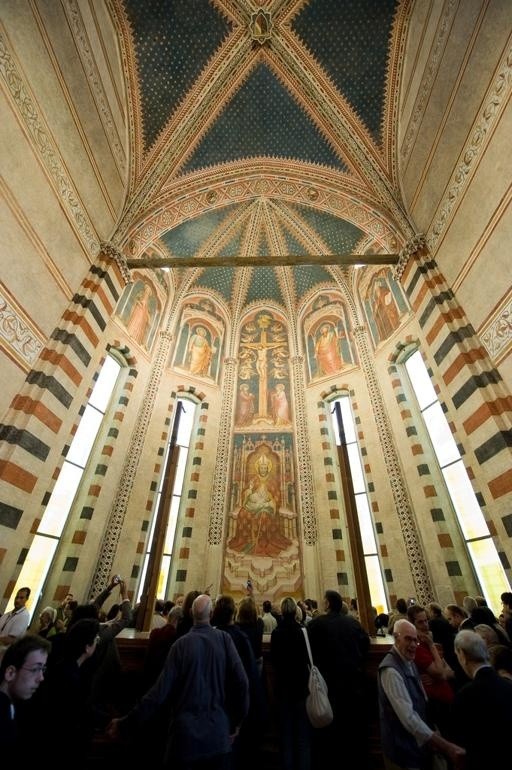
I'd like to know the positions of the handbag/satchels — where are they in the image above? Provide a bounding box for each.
[305,665,334,727]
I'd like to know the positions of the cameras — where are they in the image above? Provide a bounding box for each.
[114,576,122,582]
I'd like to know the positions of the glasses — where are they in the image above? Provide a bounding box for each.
[396,632,420,646]
[21,665,46,675]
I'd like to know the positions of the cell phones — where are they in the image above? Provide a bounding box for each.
[247,580,252,589]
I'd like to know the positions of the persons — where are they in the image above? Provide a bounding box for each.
[372,281,401,341]
[125,283,152,346]
[228,455,292,557]
[187,327,211,377]
[313,324,343,376]
[237,384,255,426]
[270,384,289,426]
